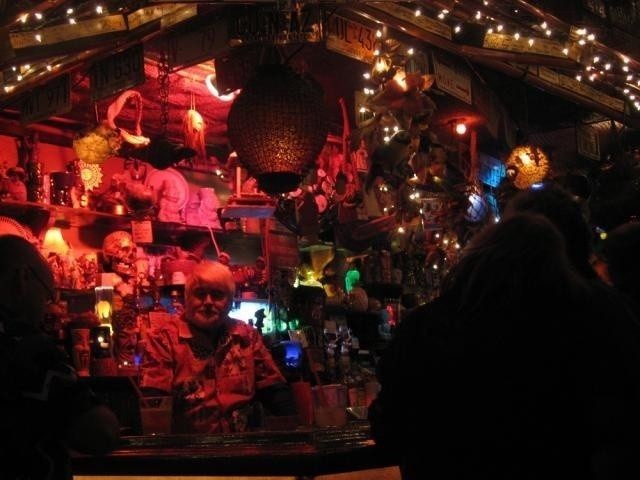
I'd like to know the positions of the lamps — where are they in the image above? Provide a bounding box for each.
[227,45,328,195]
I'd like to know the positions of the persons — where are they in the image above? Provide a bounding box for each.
[135,256,287,431]
[0,233,123,480]
[367,154,639,480]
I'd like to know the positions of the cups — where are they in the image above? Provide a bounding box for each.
[313,385,346,429]
[139,395,173,436]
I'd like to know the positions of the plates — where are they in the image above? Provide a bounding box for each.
[144,167,189,212]
